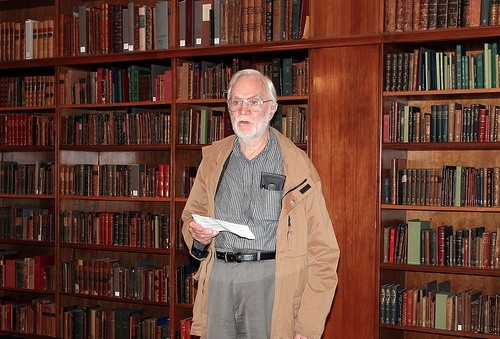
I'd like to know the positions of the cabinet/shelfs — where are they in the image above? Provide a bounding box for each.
[0,0,500,339]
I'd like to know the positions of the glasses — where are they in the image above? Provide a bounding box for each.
[227,97,273,112]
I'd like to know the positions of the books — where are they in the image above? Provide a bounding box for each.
[0,0,308,339]
[379,0,500,339]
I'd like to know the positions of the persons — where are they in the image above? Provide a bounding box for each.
[180,69,340,339]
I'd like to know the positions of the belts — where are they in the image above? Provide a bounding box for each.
[214,250,275,262]
